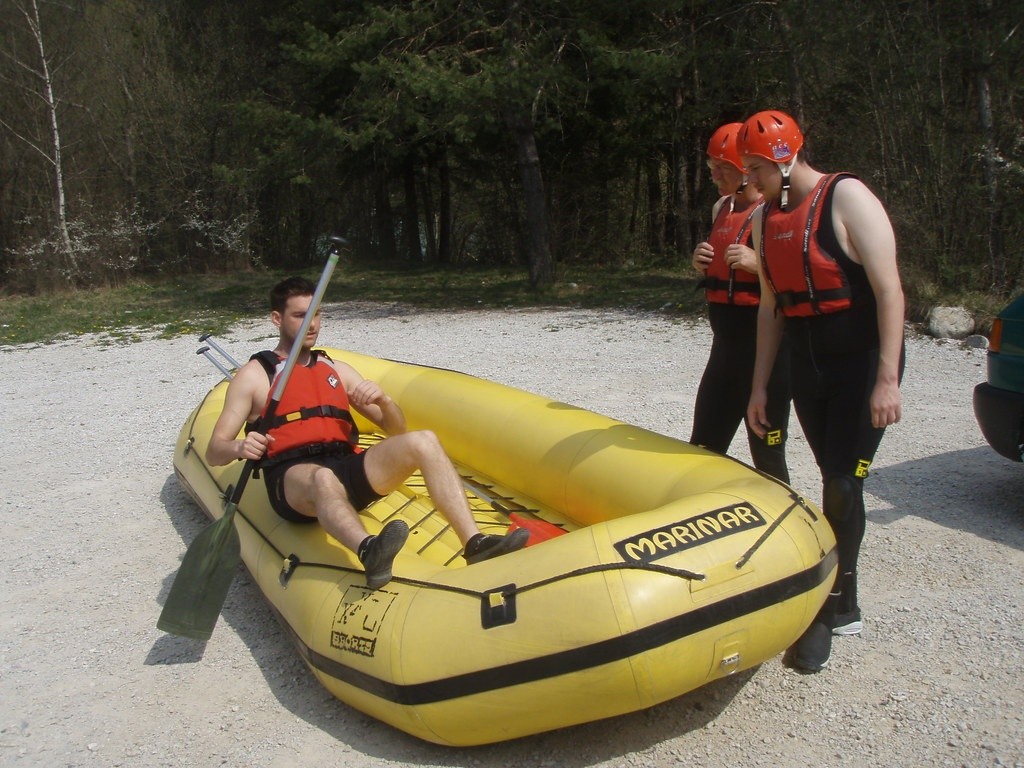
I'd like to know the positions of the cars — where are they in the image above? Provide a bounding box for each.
[974,290,1024,462]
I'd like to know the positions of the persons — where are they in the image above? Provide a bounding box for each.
[735,109,908,636]
[204,277,531,591]
[689,120,791,487]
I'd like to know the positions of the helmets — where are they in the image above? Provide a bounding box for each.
[737,110,804,162]
[707,122,750,174]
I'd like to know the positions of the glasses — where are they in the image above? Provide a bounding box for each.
[706,160,735,172]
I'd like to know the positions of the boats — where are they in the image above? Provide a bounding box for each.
[174,347,839,748]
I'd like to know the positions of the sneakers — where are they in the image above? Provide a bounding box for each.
[461,527,530,566]
[360,520,409,591]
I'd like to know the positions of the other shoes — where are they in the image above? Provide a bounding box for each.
[793,612,831,672]
[830,605,863,634]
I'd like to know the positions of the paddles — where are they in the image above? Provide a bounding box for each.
[195,328,573,550]
[155,234,351,644]
